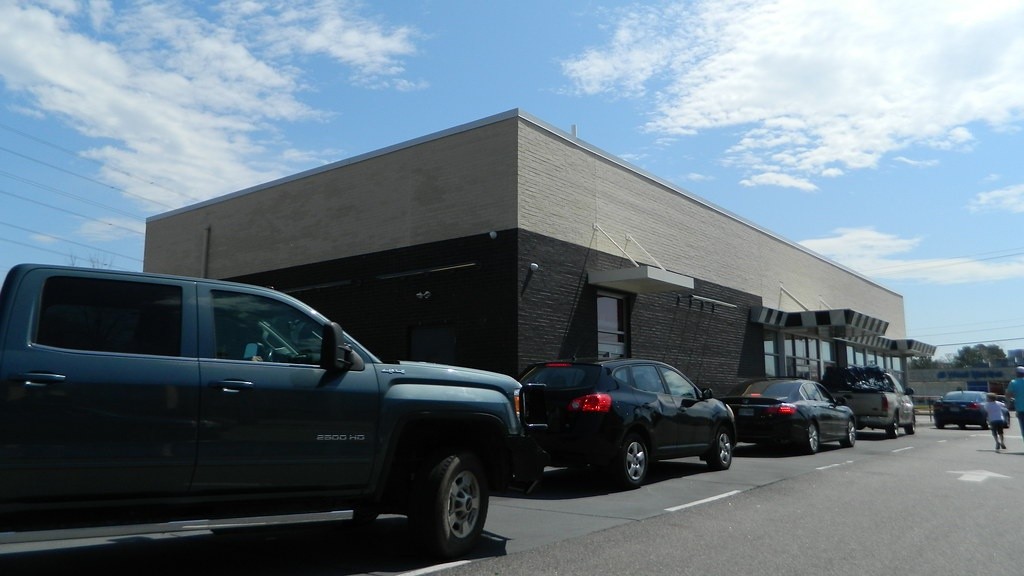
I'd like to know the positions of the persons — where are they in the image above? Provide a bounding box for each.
[983,393,1009,450]
[1006,366,1024,443]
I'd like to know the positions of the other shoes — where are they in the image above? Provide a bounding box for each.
[995,442,1001,449]
[1000,443,1007,449]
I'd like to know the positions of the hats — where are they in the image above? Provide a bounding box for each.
[1015,366,1024,373]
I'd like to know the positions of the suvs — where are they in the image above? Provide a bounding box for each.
[516,350,739,492]
[0,262,528,561]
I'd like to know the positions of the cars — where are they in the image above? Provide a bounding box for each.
[932,387,1015,430]
[831,372,916,440]
[712,376,857,456]
